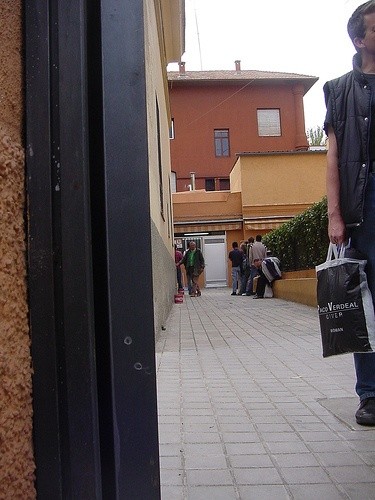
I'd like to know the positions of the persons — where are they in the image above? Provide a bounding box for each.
[323,0,375,427]
[228,236,254,295]
[253,258,282,299]
[176,241,205,297]
[174,244,185,293]
[246,234,267,296]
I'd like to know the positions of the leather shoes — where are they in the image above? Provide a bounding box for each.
[355,397,375,424]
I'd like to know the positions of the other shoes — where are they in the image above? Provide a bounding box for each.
[191,294,195,297]
[253,295,262,299]
[238,293,241,295]
[242,293,246,296]
[231,292,236,295]
[197,290,201,296]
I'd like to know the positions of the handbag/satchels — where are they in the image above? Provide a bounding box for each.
[315,239,375,357]
[241,263,246,273]
[264,284,273,298]
